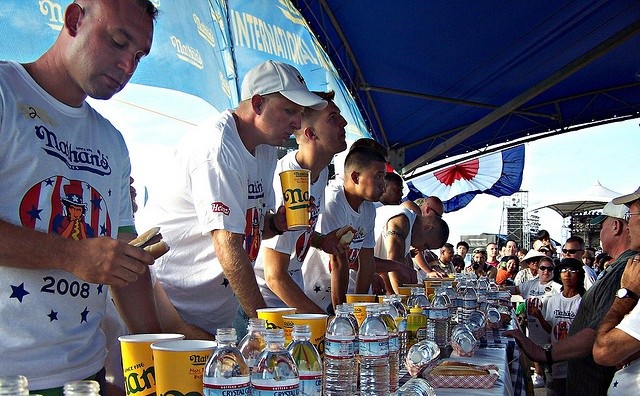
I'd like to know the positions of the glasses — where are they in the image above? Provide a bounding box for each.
[625,210,640,220]
[561,244,583,254]
[559,268,578,274]
[539,266,554,271]
[474,250,485,254]
[429,207,442,218]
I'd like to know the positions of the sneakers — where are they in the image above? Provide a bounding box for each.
[531,372,545,387]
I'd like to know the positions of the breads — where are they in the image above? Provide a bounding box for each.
[339,230,354,248]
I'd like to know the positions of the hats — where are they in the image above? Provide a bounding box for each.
[241,60,329,111]
[554,259,585,280]
[473,248,487,257]
[520,249,547,263]
[590,201,630,225]
[612,187,640,204]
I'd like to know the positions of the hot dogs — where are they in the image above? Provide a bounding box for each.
[123,227,170,260]
[432,361,489,374]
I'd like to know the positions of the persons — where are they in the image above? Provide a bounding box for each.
[99,175,215,395]
[349,137,389,160]
[455,240,470,259]
[398,199,423,216]
[514,249,542,286]
[372,172,404,209]
[302,145,386,314]
[536,229,551,243]
[505,255,520,281]
[135,59,329,334]
[231,89,348,346]
[502,201,638,395]
[517,249,528,262]
[529,257,587,346]
[466,247,498,278]
[538,244,553,257]
[485,243,500,267]
[505,239,518,256]
[347,202,449,295]
[592,186,640,396]
[582,250,595,269]
[496,242,506,263]
[412,195,449,279]
[451,254,465,273]
[519,257,563,299]
[596,252,609,267]
[0,0,163,396]
[600,255,613,271]
[562,236,597,291]
[438,242,457,274]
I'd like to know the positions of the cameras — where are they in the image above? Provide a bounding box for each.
[473,262,482,270]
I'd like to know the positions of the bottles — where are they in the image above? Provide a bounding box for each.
[375,302,401,391]
[383,293,407,369]
[323,303,359,396]
[201,328,250,396]
[250,328,300,395]
[236,317,269,372]
[451,324,476,353]
[285,324,322,396]
[360,305,392,396]
[407,286,430,352]
[408,339,441,365]
[388,379,440,396]
[430,272,501,349]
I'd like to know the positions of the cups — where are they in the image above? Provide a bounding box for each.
[279,168,313,231]
[282,313,329,355]
[149,340,218,396]
[117,332,186,396]
[255,307,297,329]
[377,294,410,306]
[345,293,376,331]
[398,286,412,296]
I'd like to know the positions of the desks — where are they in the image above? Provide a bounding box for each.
[395,350,514,396]
[478,313,528,396]
